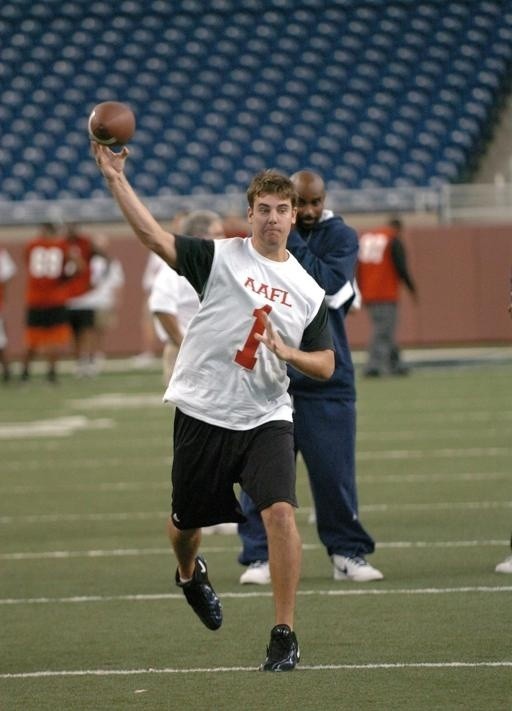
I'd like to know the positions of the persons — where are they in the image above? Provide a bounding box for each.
[494,529,512,575]
[143,211,237,535]
[1,214,120,383]
[89,141,335,673]
[237,171,383,585]
[357,219,418,376]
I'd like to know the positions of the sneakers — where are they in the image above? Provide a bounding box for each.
[172,550,226,634]
[238,560,275,586]
[256,623,305,677]
[330,552,386,586]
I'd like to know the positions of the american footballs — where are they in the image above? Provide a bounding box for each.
[87,101,136,147]
